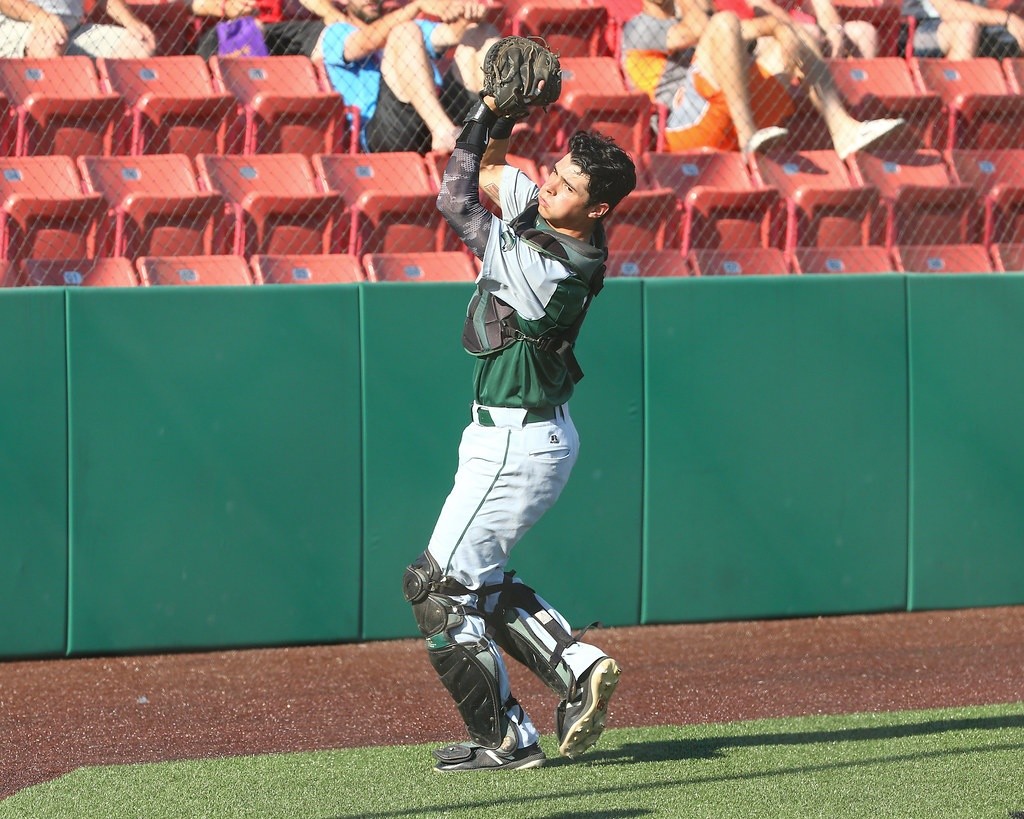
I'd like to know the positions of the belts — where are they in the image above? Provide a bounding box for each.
[478,404,563,428]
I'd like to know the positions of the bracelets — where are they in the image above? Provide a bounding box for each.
[1002,9,1013,29]
[216,1,229,16]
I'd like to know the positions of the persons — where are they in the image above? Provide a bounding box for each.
[401,54,638,772]
[901,0,1024,60]
[619,0,909,157]
[171,1,529,153]
[0,1,157,59]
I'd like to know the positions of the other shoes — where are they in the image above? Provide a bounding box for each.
[742,125,791,162]
[832,117,907,160]
[430,127,462,157]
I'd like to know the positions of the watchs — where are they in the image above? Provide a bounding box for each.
[833,22,847,33]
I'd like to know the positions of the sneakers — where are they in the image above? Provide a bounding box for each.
[432,737,548,774]
[558,657,622,760]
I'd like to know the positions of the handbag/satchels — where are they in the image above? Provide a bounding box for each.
[216,8,267,58]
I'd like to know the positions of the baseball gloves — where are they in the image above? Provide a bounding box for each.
[480,33,562,121]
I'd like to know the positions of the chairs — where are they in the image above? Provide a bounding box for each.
[0,0,1024,288]
